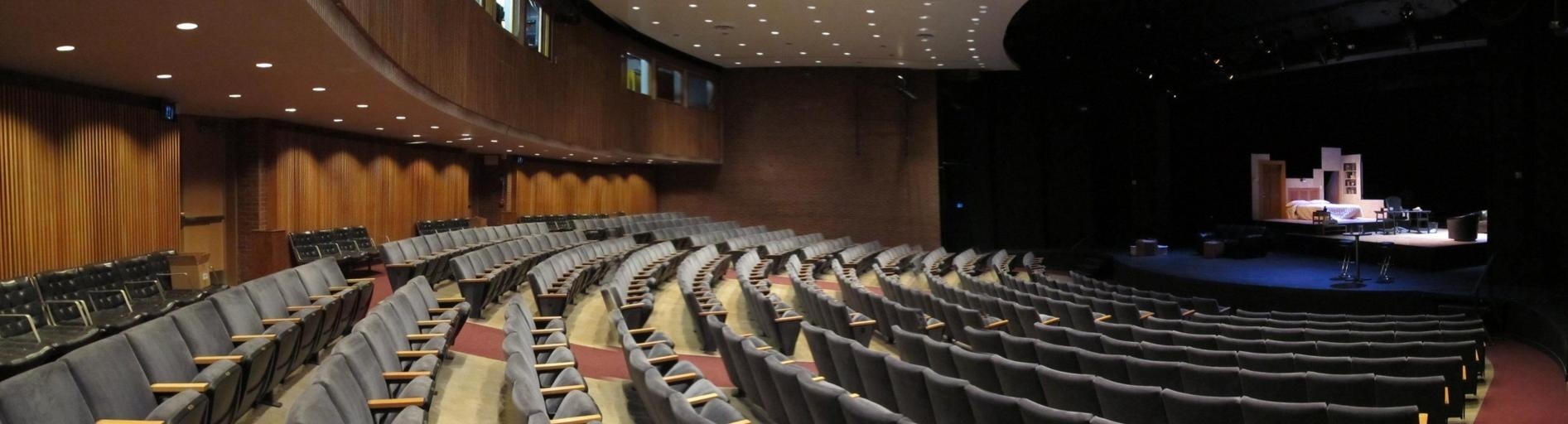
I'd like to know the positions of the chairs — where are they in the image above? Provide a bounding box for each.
[0,210,1489,424]
[1385,196,1410,233]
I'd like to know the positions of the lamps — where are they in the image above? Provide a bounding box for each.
[1301,177,1306,182]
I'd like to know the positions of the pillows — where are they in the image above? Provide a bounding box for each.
[1285,200,1308,207]
[1310,199,1330,203]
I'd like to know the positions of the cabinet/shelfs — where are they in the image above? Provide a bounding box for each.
[1343,163,1357,195]
[1259,160,1286,220]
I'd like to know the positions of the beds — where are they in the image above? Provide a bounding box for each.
[1286,203,1361,220]
